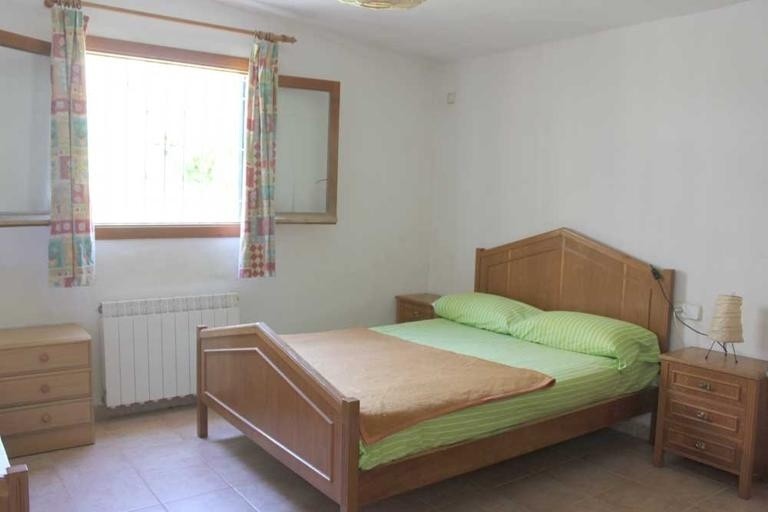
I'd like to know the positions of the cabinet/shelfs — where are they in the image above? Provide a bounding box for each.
[0,324,94,458]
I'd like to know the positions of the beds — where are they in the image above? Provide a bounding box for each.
[195,226,677,510]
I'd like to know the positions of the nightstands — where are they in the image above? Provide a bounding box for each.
[652,346,768,499]
[394,293,440,320]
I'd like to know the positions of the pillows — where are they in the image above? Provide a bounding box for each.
[431,293,540,335]
[510,310,660,370]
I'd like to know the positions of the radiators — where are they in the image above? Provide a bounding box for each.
[99,292,241,409]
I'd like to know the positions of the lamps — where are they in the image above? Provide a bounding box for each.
[705,293,744,364]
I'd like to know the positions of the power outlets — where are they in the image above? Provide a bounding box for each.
[673,304,702,320]
